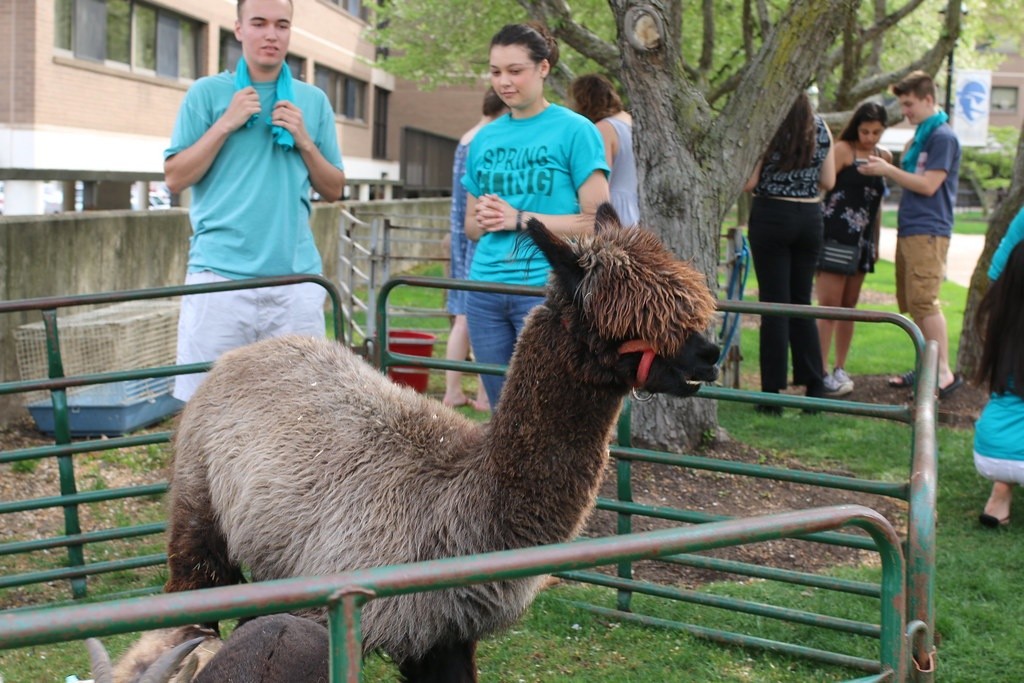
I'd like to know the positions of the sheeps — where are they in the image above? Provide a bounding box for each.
[81,613,330,683]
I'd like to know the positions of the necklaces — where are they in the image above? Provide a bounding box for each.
[873,147,877,158]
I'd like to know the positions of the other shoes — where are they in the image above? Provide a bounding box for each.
[802,408,821,414]
[754,404,784,415]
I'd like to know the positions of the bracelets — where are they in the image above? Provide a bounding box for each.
[516,209,526,232]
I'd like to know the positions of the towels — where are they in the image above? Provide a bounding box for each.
[900,110,948,173]
[234,53,296,152]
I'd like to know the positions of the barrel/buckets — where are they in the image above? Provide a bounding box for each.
[374,331,435,392]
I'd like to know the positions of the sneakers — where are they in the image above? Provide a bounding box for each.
[833,368,854,387]
[822,372,852,396]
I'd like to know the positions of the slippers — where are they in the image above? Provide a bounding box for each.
[453,395,488,413]
[889,370,916,387]
[979,514,1010,528]
[939,374,963,399]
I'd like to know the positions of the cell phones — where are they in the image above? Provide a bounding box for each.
[855,159,868,167]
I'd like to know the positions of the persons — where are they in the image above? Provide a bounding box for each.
[571,69,641,237]
[163,2,348,402]
[855,68,961,400]
[742,84,838,416]
[967,196,1024,529]
[815,102,895,400]
[438,84,512,416]
[462,24,614,428]
